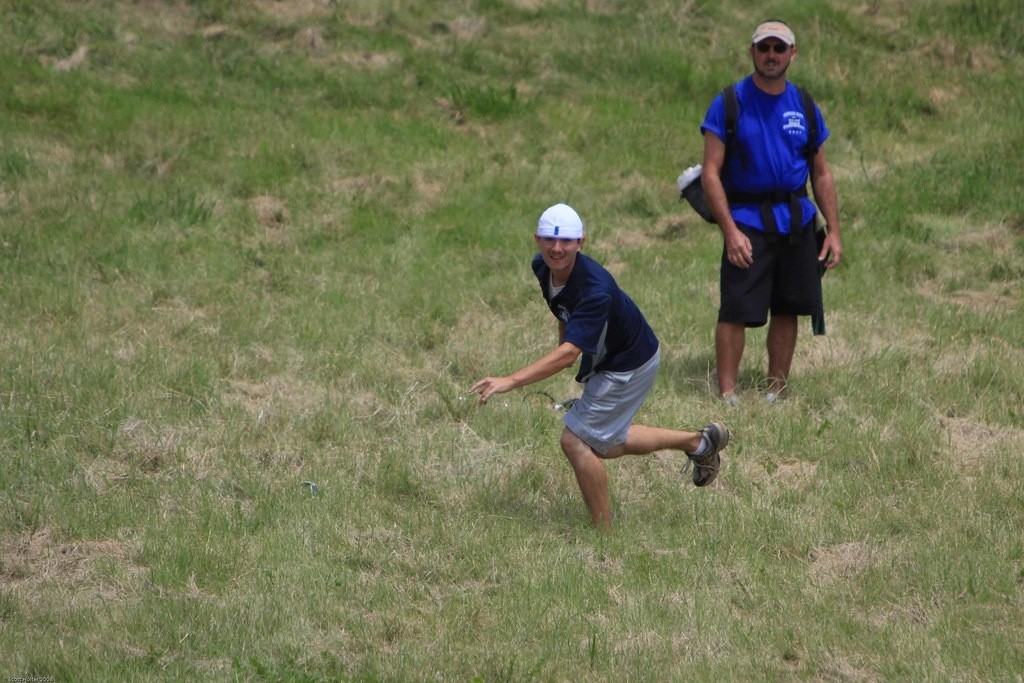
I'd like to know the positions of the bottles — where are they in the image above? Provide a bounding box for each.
[677,163,705,193]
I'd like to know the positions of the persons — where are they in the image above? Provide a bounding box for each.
[701,19,843,412]
[468,202,729,538]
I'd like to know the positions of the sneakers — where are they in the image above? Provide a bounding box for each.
[767,394,785,400]
[686,420,729,486]
[719,391,738,405]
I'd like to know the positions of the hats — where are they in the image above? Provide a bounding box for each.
[537,205,583,239]
[752,22,795,46]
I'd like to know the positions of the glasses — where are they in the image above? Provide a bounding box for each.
[753,44,792,53]
